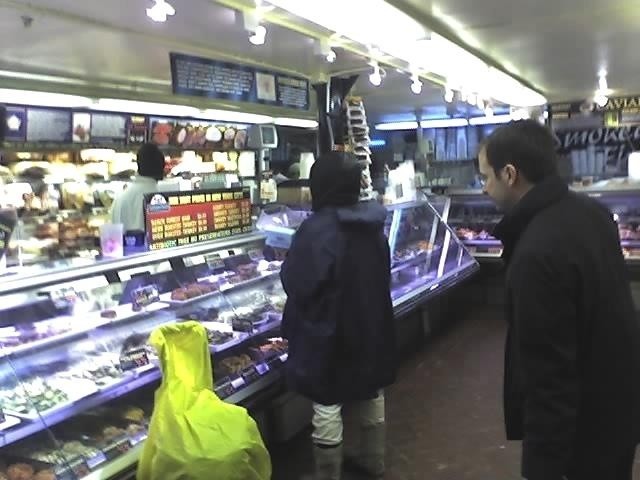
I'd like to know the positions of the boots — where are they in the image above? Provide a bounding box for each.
[344,423,386,477]
[299,445,343,480]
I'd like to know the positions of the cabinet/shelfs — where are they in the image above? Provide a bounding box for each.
[0,196,482,480]
[441,187,639,304]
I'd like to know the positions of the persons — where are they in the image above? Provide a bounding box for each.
[475,117,640,478]
[276,144,397,480]
[108,141,166,236]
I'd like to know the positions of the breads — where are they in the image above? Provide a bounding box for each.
[0,462,55,480]
[171,284,216,300]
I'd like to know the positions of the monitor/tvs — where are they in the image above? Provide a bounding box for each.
[247,125,278,149]
